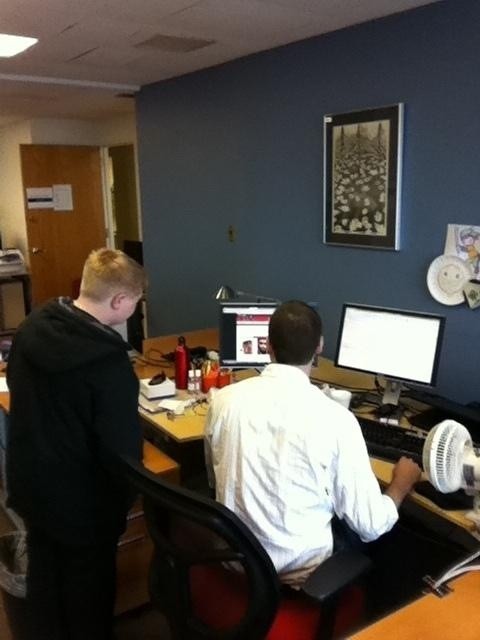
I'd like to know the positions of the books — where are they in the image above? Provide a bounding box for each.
[139,394,178,413]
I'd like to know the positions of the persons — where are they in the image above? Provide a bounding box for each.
[257,336,268,355]
[6,248,148,640]
[203,300,422,630]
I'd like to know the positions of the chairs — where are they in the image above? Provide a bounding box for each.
[110,448,389,629]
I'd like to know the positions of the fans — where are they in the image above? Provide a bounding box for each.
[421,418,480,533]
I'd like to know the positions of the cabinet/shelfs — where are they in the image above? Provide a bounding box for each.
[1,272,33,335]
[115,489,181,616]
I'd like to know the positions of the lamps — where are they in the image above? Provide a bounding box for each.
[210,282,284,314]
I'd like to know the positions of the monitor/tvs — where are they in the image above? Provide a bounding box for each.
[335,303,447,389]
[219,302,318,368]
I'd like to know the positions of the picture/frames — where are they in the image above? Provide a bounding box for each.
[318,100,405,252]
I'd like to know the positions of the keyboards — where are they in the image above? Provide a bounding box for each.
[355,417,428,472]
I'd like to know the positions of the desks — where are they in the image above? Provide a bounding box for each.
[1,390,180,484]
[344,569,480,640]
[125,326,480,543]
